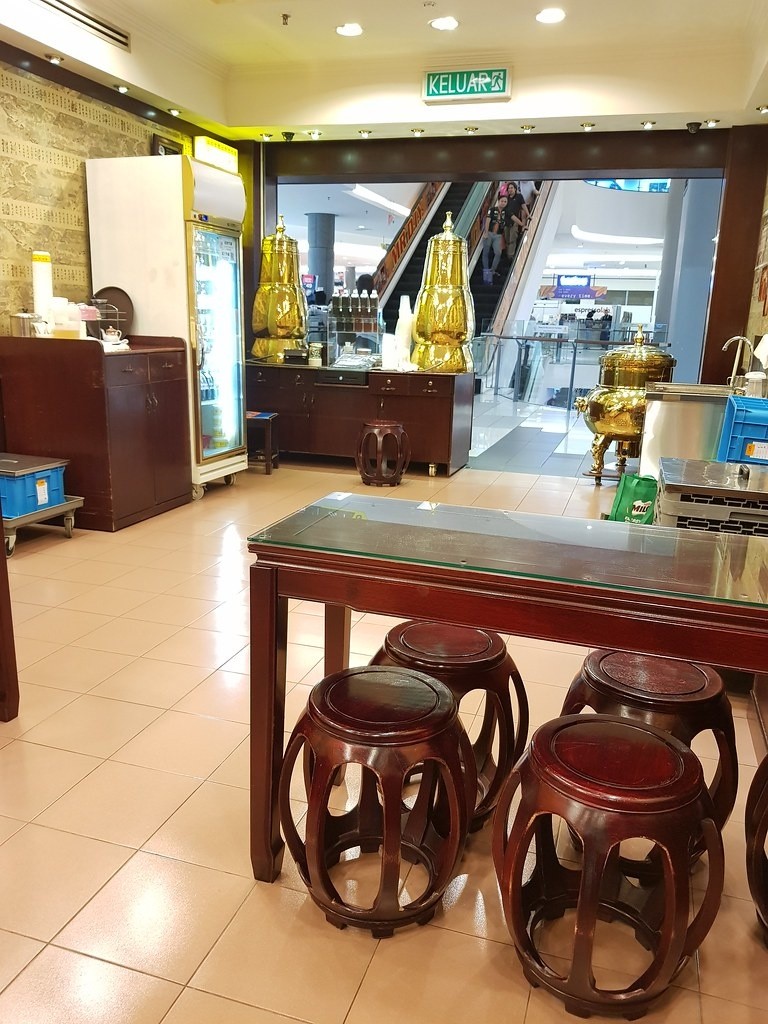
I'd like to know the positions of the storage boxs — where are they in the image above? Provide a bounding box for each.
[0,466,67,519]
[715,395,768,466]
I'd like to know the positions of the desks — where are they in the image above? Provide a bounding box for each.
[651,458,768,537]
[248,492,768,885]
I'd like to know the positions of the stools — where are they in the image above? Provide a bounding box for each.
[558,649,739,879]
[246,411,279,476]
[278,666,477,939]
[356,420,412,487]
[745,752,768,950]
[492,715,727,1022]
[368,620,529,834]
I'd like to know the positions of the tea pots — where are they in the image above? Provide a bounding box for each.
[100,326,122,342]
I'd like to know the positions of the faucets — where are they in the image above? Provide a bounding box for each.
[721,333,753,374]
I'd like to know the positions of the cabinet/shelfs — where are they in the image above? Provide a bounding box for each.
[246,358,481,478]
[0,334,191,533]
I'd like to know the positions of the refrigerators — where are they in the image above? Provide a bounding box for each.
[85,155,249,500]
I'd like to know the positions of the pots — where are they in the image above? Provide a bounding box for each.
[9,308,46,338]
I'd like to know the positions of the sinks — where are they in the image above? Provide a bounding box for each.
[658,386,733,394]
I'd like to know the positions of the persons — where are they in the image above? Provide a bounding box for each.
[600,311,612,351]
[582,312,594,349]
[356,274,373,295]
[482,180,542,285]
[314,291,326,305]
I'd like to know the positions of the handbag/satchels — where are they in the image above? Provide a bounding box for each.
[606,474,658,527]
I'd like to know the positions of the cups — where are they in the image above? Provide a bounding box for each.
[727,376,745,386]
[90,299,108,318]
[357,348,372,355]
[398,295,411,370]
